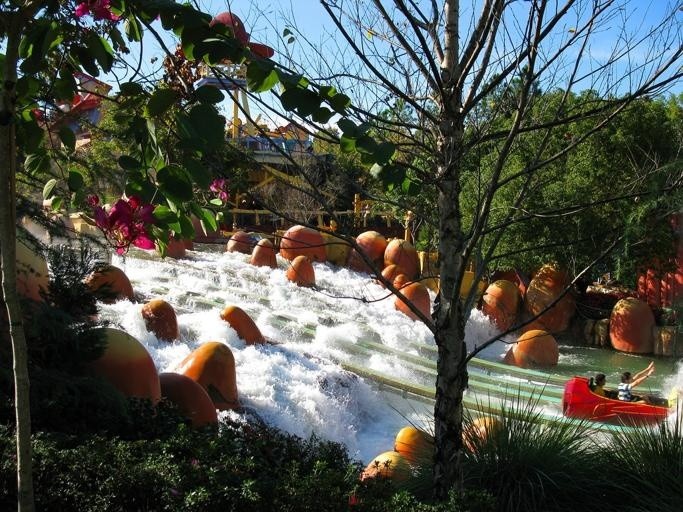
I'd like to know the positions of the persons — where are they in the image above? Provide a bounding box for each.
[616,360,657,405]
[592,370,608,397]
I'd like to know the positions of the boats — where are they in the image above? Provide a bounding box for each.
[560,375,682,431]
